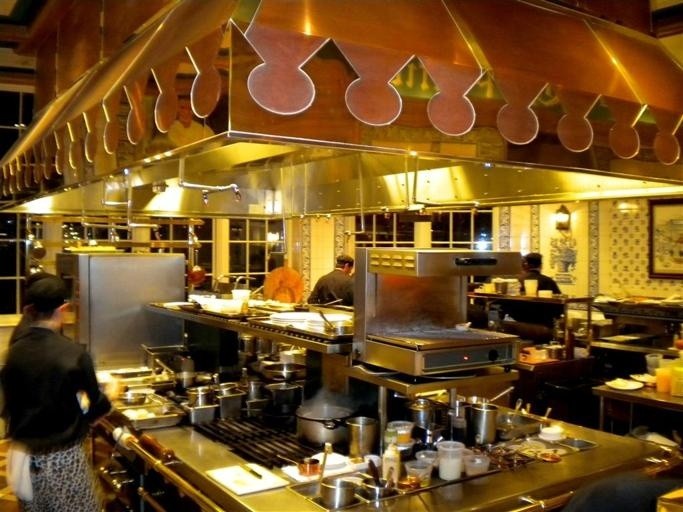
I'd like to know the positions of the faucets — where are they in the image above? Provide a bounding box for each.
[211,272,238,293]
[228,274,256,295]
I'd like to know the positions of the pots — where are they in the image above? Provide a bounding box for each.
[292,405,354,450]
[174,372,203,389]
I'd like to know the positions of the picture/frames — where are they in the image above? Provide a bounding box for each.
[646,196,682,281]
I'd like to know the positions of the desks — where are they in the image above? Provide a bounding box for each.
[591,377,682,433]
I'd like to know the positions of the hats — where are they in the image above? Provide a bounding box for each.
[337,255,354,265]
[522,253,542,268]
[28,278,65,306]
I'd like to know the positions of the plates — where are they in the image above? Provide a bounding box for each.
[603,373,656,392]
[270,311,351,330]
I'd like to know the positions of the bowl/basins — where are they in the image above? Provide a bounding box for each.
[114,392,148,405]
[262,382,302,414]
[261,364,305,382]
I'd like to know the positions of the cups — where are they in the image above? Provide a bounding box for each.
[450,398,499,445]
[184,383,239,405]
[231,290,250,306]
[437,483,462,504]
[391,420,413,444]
[483,283,493,293]
[344,415,375,458]
[321,477,393,510]
[645,351,682,397]
[413,221,432,249]
[405,441,491,486]
[495,282,506,294]
[524,279,537,297]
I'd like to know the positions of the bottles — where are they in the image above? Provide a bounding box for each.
[381,421,401,488]
[238,334,281,362]
[238,366,249,385]
[136,435,175,462]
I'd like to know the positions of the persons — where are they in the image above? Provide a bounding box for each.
[7,272,69,348]
[168,94,214,148]
[0,273,112,512]
[502,252,564,342]
[306,254,355,309]
